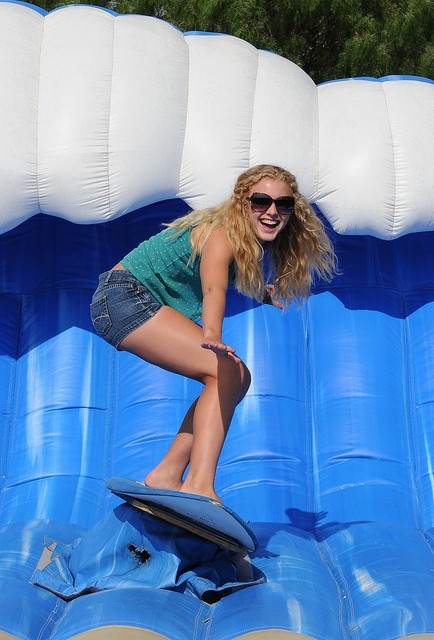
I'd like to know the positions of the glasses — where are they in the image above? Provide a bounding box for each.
[243,192,298,215]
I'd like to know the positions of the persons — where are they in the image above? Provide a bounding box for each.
[89,164,343,505]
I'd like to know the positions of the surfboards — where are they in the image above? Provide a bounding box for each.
[106,479,259,553]
